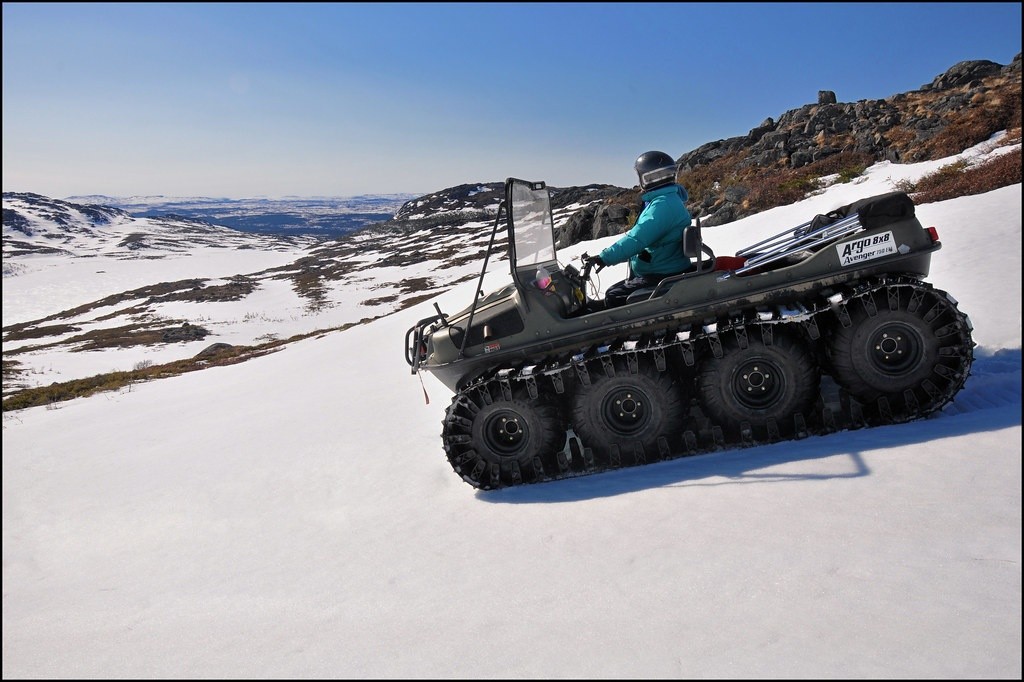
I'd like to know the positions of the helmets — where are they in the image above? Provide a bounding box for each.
[634,150,678,191]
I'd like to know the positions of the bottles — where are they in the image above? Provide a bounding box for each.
[536,264,556,297]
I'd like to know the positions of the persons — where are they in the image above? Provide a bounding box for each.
[582,151,691,309]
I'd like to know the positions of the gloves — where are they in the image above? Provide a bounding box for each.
[581,254,606,274]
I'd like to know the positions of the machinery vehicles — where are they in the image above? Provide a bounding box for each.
[403,176,979,492]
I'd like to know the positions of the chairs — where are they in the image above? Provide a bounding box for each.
[626,217,717,306]
